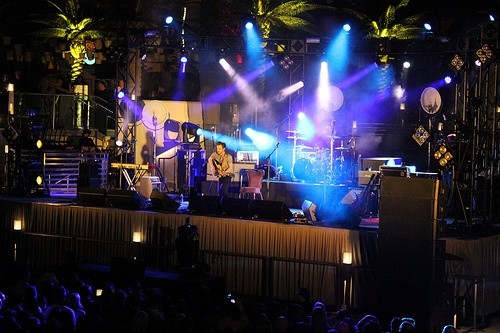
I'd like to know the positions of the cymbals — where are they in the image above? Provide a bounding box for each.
[286,130,303,133]
[287,136,307,140]
[335,147,349,149]
[325,135,341,138]
[287,149,300,150]
[293,145,313,149]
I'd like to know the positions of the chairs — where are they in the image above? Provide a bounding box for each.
[239,169,266,199]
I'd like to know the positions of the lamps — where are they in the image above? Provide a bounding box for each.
[446,53,465,72]
[161,13,175,28]
[115,140,124,147]
[117,90,131,103]
[215,51,225,64]
[181,120,202,150]
[433,145,455,169]
[87,53,95,65]
[244,15,253,30]
[424,20,435,31]
[279,55,296,71]
[410,124,431,146]
[140,47,147,60]
[474,44,498,65]
[180,50,189,63]
[164,119,182,141]
[489,11,500,22]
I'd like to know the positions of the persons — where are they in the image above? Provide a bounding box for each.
[441,325,458,333]
[93,81,116,135]
[79,129,98,179]
[114,80,129,97]
[208,141,234,198]
[0,262,417,333]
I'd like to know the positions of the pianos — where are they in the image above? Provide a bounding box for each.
[111,163,157,191]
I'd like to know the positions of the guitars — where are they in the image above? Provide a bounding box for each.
[350,174,380,217]
[211,164,235,179]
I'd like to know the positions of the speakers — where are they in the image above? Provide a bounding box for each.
[189,195,224,215]
[77,187,108,205]
[150,192,181,212]
[104,191,143,210]
[223,197,253,218]
[252,199,294,221]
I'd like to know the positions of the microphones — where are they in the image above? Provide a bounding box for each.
[275,142,279,148]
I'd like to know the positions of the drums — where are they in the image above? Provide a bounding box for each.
[292,157,319,179]
[316,148,330,159]
[302,153,317,158]
